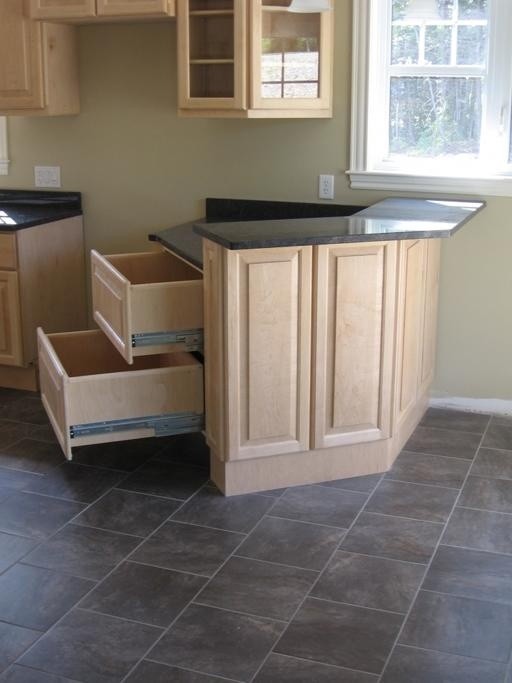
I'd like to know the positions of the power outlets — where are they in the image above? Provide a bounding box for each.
[318,173,336,199]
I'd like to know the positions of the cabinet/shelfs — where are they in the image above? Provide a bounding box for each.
[171,0,335,124]
[1,0,80,117]
[1,186,91,397]
[27,0,177,28]
[28,197,486,501]
